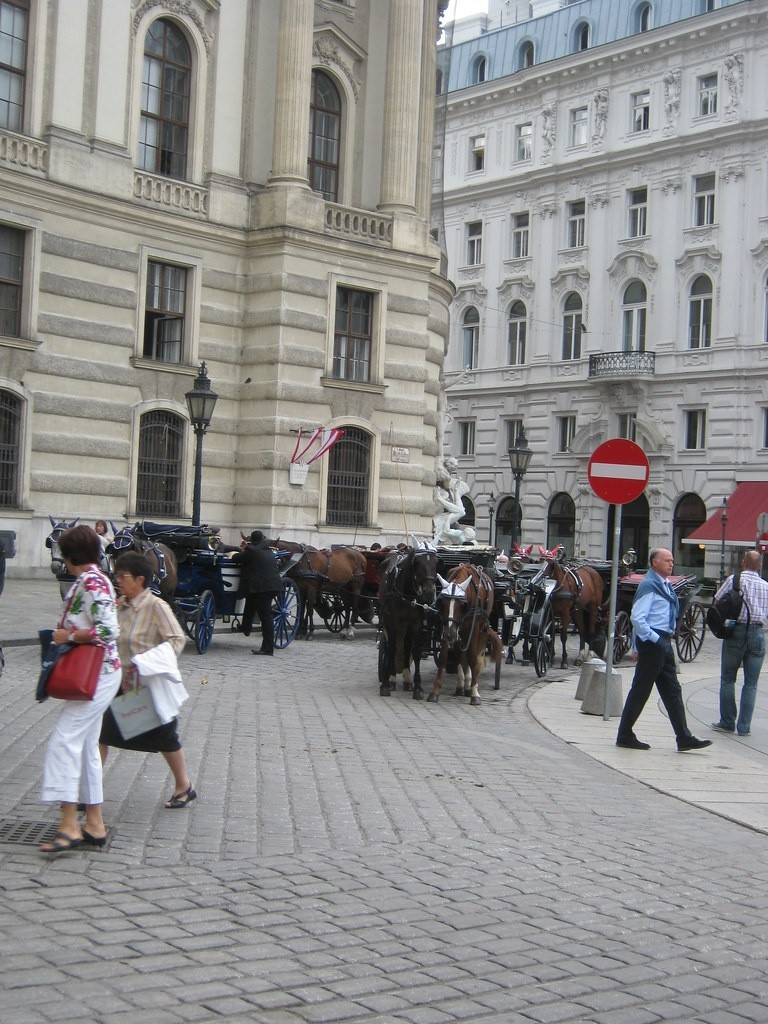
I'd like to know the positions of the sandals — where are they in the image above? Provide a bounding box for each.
[165,784,197,809]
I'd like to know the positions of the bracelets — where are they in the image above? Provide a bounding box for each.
[68,633,74,643]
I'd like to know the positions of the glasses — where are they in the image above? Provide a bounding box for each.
[113,571,135,579]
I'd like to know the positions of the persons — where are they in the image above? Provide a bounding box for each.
[541,104,553,145]
[616,549,713,751]
[210,527,242,552]
[663,71,678,121]
[59,551,198,813]
[498,555,508,563]
[592,91,607,138]
[232,531,284,656]
[724,56,739,98]
[711,550,768,736]
[436,476,478,545]
[40,524,123,851]
[95,520,113,543]
[350,543,381,623]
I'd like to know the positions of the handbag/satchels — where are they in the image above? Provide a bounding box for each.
[35,625,105,703]
[109,668,164,740]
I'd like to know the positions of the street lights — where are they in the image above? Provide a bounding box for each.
[716,494,731,587]
[506,424,535,562]
[486,491,498,547]
[183,360,219,526]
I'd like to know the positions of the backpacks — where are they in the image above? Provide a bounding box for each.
[706,572,751,640]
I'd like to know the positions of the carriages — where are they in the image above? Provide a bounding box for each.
[199,522,707,707]
[44,514,302,655]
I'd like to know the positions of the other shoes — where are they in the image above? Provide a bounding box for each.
[738,730,752,736]
[711,721,735,735]
[237,622,250,637]
[251,649,273,656]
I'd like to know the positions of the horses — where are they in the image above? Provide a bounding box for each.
[46,513,114,602]
[531,545,604,670]
[375,534,438,699]
[266,537,366,641]
[239,529,318,554]
[510,542,540,579]
[427,563,494,704]
[104,520,177,608]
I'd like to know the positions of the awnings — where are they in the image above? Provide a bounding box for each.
[681,481,768,547]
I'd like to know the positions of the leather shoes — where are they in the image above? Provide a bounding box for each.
[617,738,650,748]
[678,738,712,751]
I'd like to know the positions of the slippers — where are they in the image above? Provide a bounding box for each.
[41,827,87,852]
[78,827,106,846]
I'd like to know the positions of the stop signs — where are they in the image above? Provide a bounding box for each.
[587,438,650,505]
[757,532,768,555]
